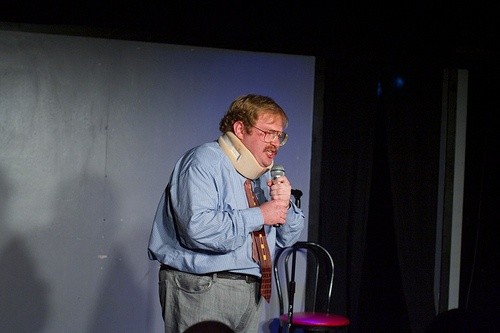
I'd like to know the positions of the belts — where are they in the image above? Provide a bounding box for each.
[165,265,261,283]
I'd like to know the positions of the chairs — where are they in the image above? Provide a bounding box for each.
[273,239,350,333]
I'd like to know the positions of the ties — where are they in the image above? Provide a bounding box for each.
[244,178,273,304]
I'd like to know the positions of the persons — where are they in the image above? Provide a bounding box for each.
[147,95,305,333]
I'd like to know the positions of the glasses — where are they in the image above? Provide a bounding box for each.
[250,124,289,146]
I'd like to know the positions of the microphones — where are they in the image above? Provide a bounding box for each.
[271,166,285,227]
